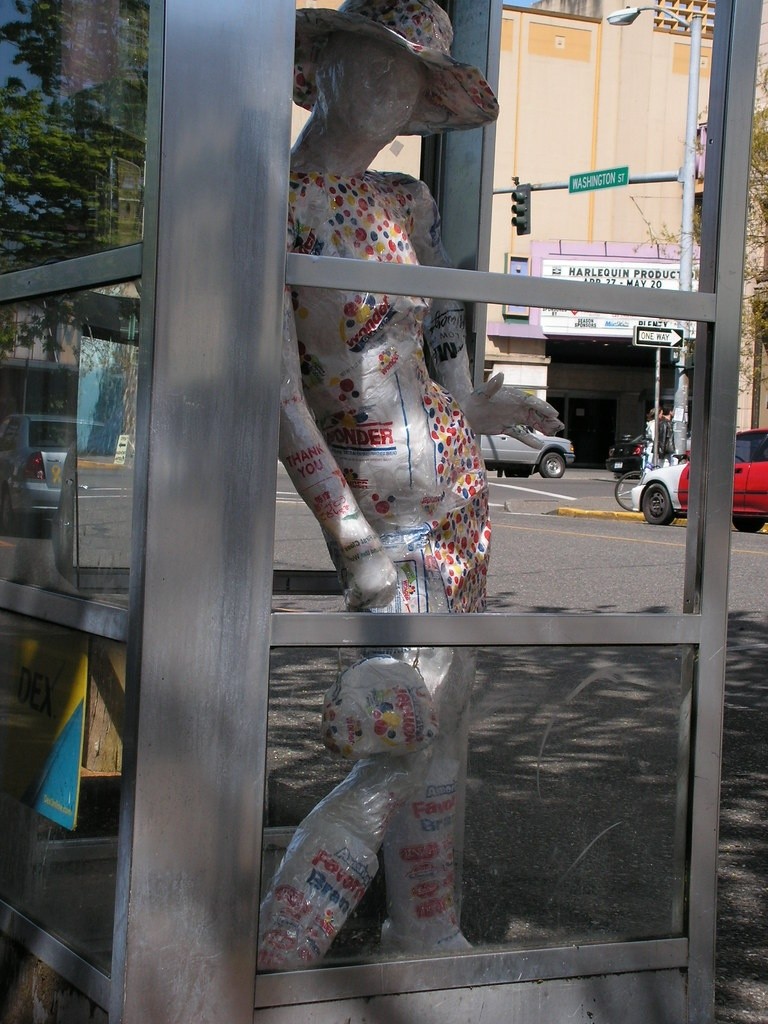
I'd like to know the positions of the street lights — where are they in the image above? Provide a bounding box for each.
[606,3,703,456]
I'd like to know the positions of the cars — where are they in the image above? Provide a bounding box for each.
[605,431,649,478]
[0,413,109,539]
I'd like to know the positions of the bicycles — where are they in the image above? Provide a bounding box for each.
[614,436,688,513]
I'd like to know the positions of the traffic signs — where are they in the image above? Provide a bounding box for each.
[631,324,686,350]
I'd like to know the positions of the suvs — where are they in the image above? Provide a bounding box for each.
[480,424,576,478]
[631,427,768,534]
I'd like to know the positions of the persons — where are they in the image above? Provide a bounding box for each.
[642,404,675,472]
[254,0,566,970]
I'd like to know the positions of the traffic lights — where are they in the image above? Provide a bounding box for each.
[510,182,531,236]
